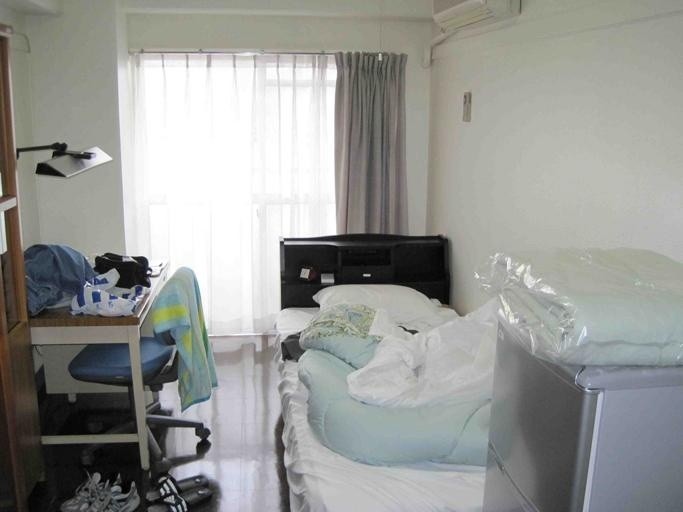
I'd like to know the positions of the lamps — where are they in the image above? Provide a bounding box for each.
[16,141,113,180]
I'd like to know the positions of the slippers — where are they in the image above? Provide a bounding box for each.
[145,474,211,512]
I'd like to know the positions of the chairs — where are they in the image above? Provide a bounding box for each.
[67,265,210,478]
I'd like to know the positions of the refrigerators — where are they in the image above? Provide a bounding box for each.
[482,316,683,511]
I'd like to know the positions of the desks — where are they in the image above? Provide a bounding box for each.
[28,259,171,471]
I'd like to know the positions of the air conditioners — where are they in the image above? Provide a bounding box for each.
[433,0,521,31]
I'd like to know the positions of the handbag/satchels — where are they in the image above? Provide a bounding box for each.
[94,252,152,287]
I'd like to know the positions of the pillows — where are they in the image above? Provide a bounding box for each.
[311,284,440,315]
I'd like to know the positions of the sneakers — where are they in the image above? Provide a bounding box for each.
[88,479,141,511]
[60,470,122,511]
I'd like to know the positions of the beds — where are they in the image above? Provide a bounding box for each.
[278,232,485,512]
